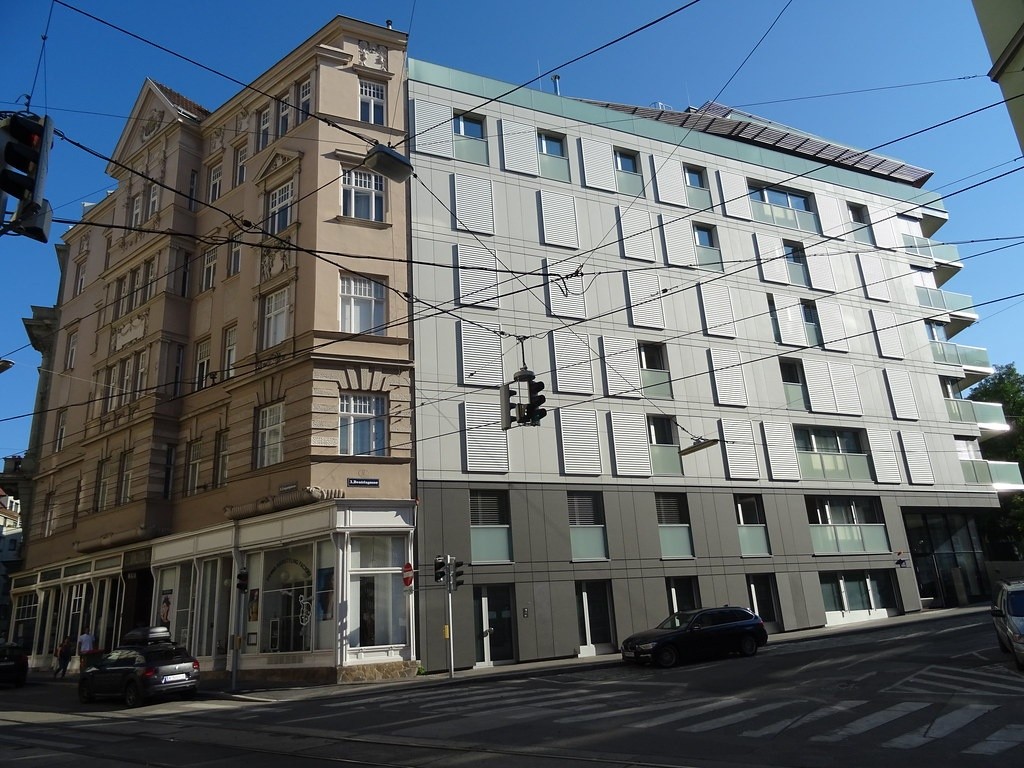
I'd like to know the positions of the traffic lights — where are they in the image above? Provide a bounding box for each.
[500,384,517,430]
[450,557,464,592]
[528,377,547,426]
[236,571,248,594]
[435,556,446,583]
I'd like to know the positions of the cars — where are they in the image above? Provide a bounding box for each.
[620,606,768,668]
[0,642,28,688]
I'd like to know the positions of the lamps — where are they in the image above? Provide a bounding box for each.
[678,439,721,456]
[363,144,414,185]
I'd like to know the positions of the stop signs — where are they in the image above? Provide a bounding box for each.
[403,564,414,586]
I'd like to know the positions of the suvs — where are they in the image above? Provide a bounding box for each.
[78,639,200,708]
[991,577,1024,671]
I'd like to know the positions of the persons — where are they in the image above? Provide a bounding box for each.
[78,626,96,655]
[52,636,74,681]
[159,597,170,631]
